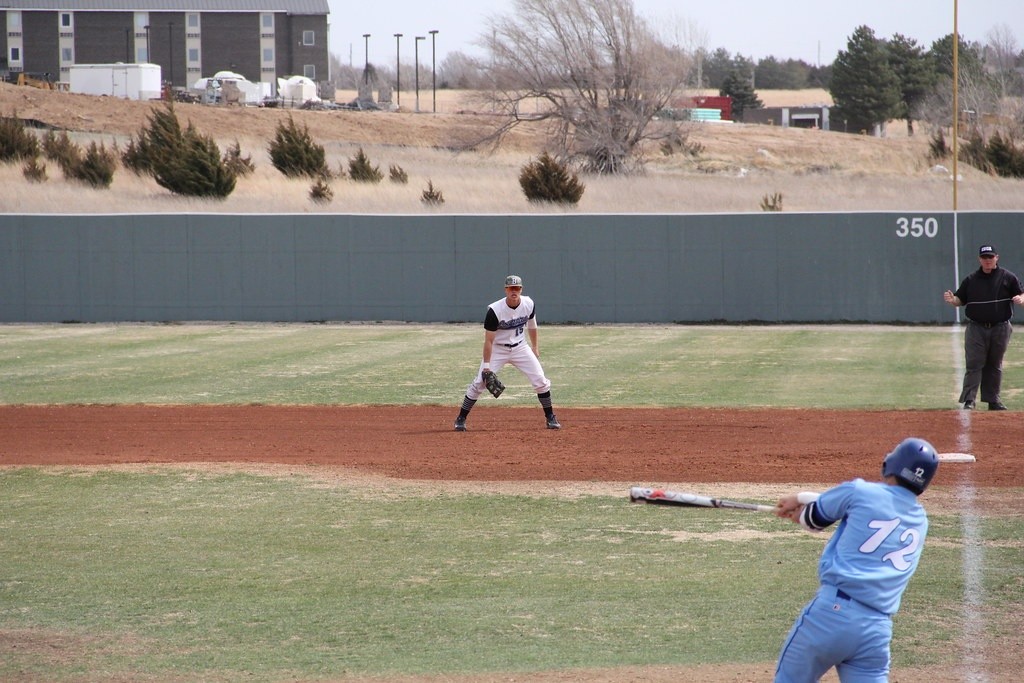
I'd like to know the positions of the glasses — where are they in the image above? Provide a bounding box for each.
[980,255,994,259]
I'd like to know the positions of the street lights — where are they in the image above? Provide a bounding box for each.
[394,33,404,106]
[143,25,152,63]
[415,37,426,112]
[363,34,372,85]
[125,27,132,64]
[167,22,175,86]
[429,30,440,112]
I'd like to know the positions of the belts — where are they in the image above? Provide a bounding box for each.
[971,321,1008,328]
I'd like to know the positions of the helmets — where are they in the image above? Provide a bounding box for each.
[882,438,938,489]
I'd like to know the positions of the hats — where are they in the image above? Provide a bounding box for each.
[504,275,522,288]
[979,243,997,257]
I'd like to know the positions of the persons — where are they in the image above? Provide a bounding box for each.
[943,245,1024,410]
[775,438,938,683]
[454,275,561,431]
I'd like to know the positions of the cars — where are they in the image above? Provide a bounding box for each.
[174,89,222,104]
[259,95,279,107]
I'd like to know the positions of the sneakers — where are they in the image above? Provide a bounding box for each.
[454,417,466,431]
[546,414,560,429]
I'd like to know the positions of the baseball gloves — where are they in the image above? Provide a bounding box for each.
[481,370,506,399]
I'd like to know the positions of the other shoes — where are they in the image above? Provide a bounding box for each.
[964,400,975,409]
[989,403,1007,410]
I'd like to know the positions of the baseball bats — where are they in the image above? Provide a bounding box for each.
[629,485,794,519]
